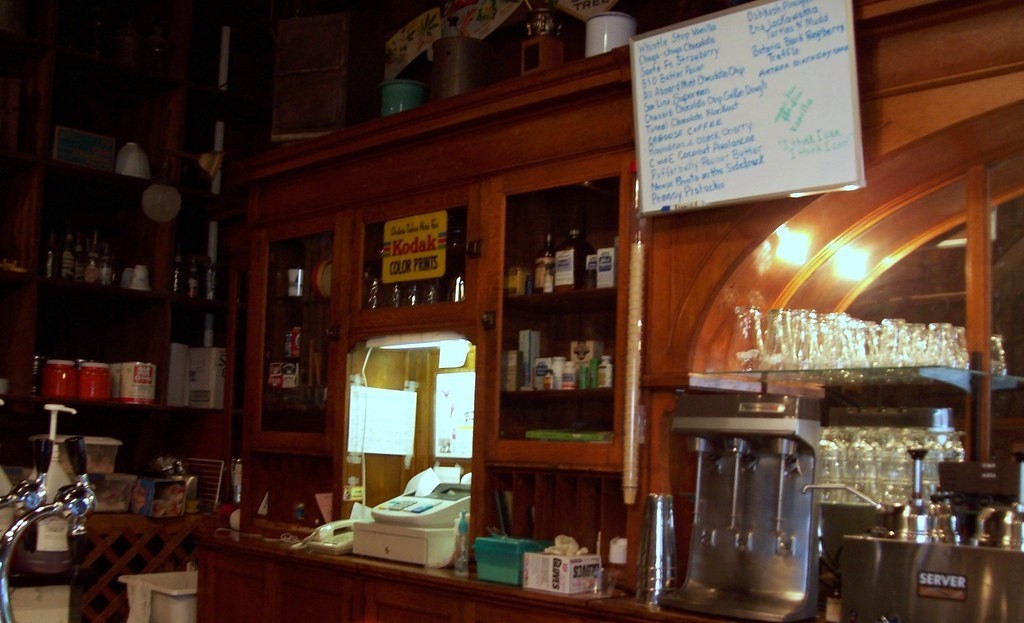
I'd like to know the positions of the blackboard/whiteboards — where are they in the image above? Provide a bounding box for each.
[627,0,867,218]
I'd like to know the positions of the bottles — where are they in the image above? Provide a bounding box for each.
[46,222,119,285]
[173,256,184,293]
[187,257,199,299]
[455,510,469,576]
[555,225,586,291]
[535,234,556,292]
[272,272,286,297]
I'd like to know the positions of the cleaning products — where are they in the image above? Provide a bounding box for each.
[36,403,84,553]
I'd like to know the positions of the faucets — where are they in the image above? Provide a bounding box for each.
[802,483,883,513]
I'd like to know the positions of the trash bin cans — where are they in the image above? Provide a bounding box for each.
[117,570,198,623]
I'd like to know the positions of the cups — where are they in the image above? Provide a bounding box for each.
[43,360,76,399]
[820,425,964,503]
[378,80,430,116]
[585,12,637,57]
[637,493,684,602]
[128,265,151,290]
[78,363,113,400]
[732,306,1004,384]
[120,268,134,288]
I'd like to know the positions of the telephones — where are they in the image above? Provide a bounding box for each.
[307,520,354,555]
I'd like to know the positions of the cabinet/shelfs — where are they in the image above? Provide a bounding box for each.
[0,0,239,623]
[190,0,1024,623]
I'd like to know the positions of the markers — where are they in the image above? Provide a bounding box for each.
[660,201,702,211]
